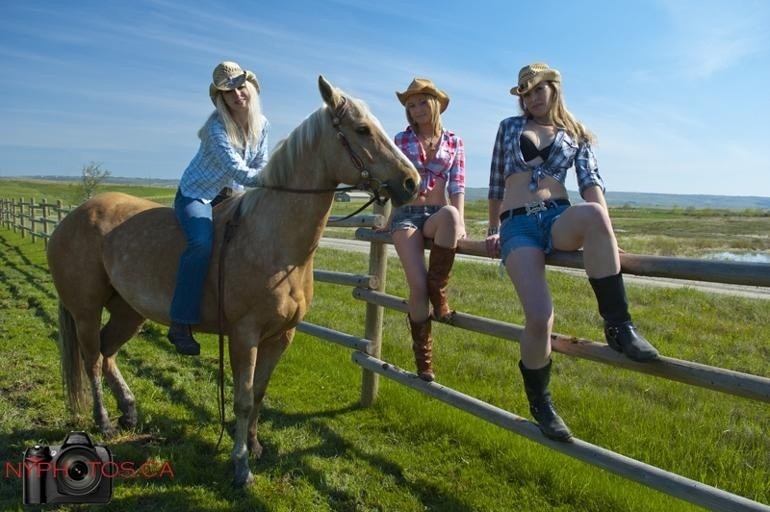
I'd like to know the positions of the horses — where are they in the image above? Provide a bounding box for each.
[44,75,421,494]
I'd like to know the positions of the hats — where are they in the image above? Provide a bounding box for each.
[510,64,561,95]
[396,78,448,114]
[210,61,260,107]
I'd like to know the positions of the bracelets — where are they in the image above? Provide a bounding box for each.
[487,224,498,237]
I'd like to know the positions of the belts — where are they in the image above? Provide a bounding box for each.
[500,198,570,222]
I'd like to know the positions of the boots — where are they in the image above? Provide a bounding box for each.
[518,359,573,441]
[427,244,457,322]
[589,270,659,361]
[408,312,435,381]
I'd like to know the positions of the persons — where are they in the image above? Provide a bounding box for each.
[167,62,269,355]
[486,62,659,440]
[372,79,466,381]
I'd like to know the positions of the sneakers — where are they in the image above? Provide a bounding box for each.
[168,327,200,355]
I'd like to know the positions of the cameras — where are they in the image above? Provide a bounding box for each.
[20,429,114,508]
